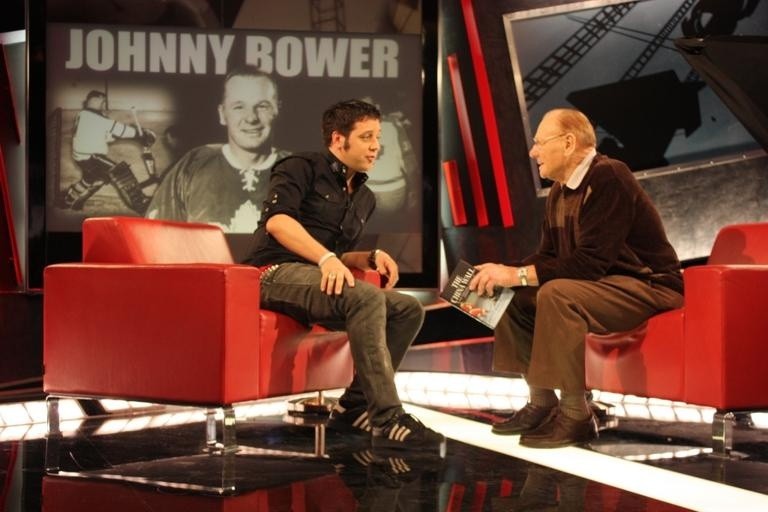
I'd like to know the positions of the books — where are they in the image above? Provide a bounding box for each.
[438,260,516,332]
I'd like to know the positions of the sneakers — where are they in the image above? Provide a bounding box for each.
[372,414,443,450]
[326,402,371,435]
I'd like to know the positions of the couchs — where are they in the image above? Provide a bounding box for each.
[43,218,381,496]
[586,222,766,462]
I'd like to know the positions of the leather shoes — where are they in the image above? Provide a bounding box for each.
[492,403,551,435]
[519,406,600,449]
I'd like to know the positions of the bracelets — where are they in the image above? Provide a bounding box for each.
[368,248,380,270]
[318,252,336,266]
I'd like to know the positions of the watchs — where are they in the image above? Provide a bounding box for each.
[518,266,527,286]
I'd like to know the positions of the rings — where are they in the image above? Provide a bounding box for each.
[328,271,336,279]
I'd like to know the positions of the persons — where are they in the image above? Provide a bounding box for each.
[57,90,156,214]
[352,95,423,244]
[238,98,444,451]
[158,125,205,186]
[145,65,294,234]
[468,108,686,449]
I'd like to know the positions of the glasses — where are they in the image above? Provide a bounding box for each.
[533,135,567,146]
